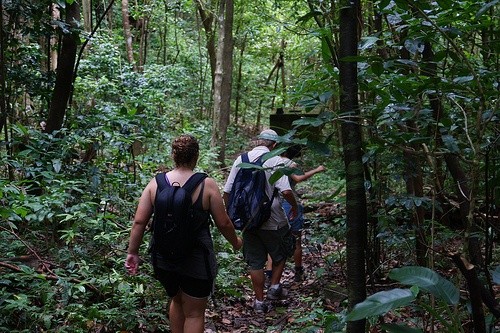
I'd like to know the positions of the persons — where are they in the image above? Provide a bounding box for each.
[123,132,244,333]
[220,130,300,313]
[267,143,327,289]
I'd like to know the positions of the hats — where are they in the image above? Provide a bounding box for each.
[258,129,278,141]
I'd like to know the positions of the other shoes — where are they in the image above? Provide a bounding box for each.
[294,267,304,281]
[266,285,290,301]
[254,298,269,313]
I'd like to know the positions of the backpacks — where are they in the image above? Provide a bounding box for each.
[226,151,277,230]
[153,171,209,256]
